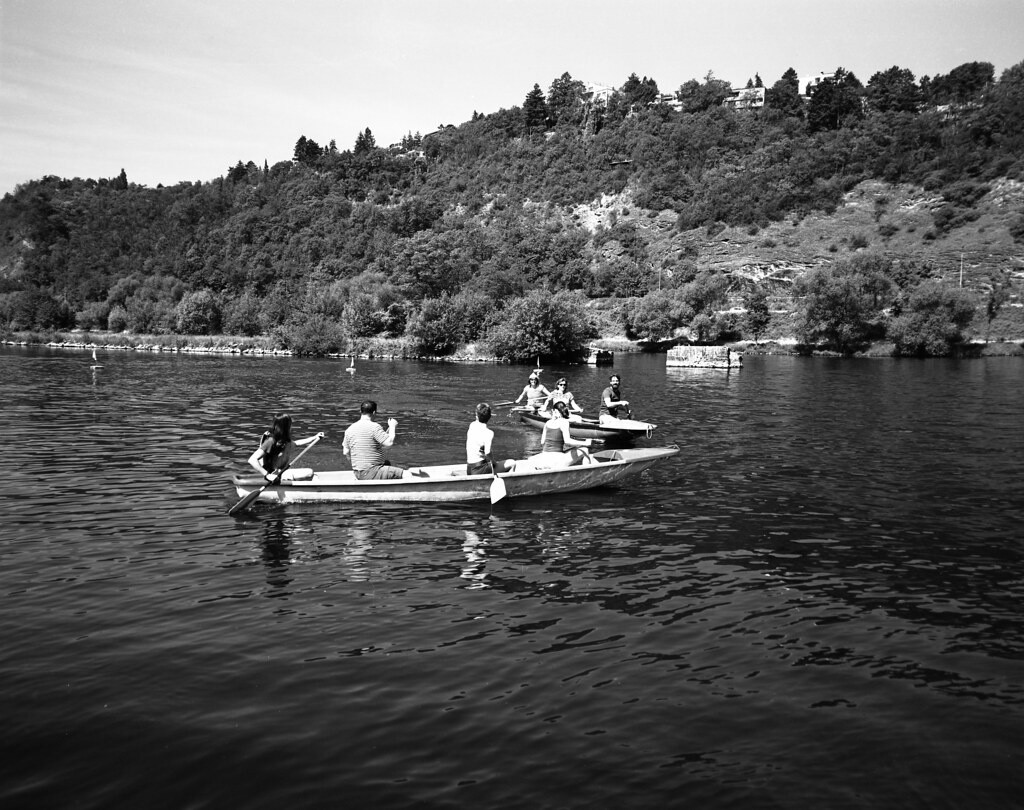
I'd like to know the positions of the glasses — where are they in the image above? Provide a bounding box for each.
[559,384,566,386]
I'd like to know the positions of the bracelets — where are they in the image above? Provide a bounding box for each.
[264,472,270,480]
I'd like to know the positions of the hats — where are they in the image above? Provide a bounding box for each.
[529,372,539,378]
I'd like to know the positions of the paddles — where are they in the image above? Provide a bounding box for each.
[564,446,574,453]
[475,403,507,505]
[625,404,632,420]
[492,396,548,406]
[229,433,322,516]
[386,427,389,433]
[510,405,580,413]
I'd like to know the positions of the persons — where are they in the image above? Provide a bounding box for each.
[514,373,584,422]
[599,374,630,424]
[341,400,412,480]
[540,401,593,468]
[247,412,325,481]
[464,403,517,475]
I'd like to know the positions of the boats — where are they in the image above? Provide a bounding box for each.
[225,443,681,508]
[517,403,659,443]
[662,343,744,370]
